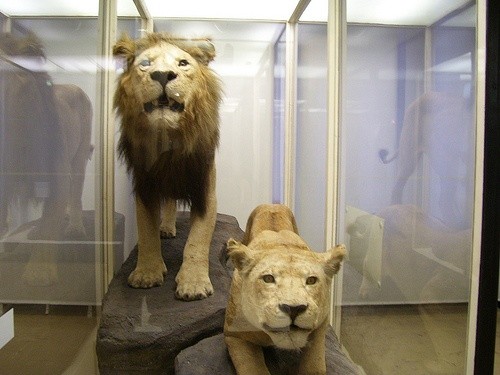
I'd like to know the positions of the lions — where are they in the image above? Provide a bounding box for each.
[111,29,227,301]
[0,30,95,286]
[218,203,348,375]
